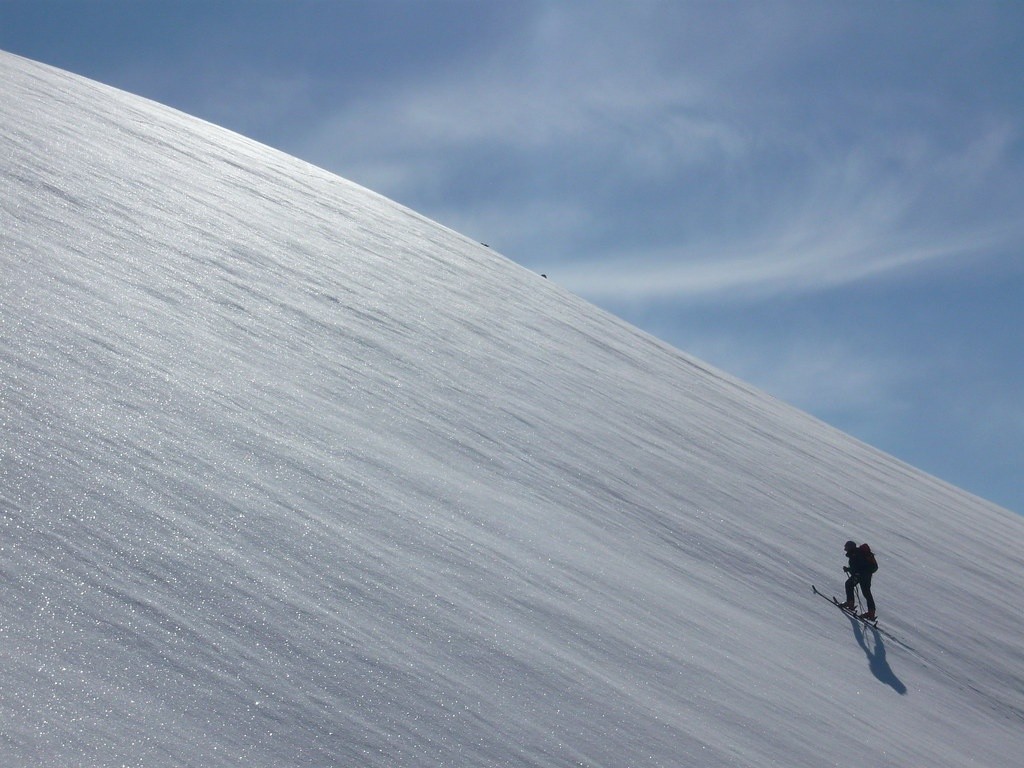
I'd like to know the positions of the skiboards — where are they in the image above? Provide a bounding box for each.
[811,584,878,628]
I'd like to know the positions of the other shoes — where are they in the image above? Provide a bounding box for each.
[863,610,875,618]
[842,600,854,607]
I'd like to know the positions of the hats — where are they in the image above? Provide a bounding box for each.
[845,541,856,550]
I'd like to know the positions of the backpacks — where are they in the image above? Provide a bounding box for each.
[859,543,878,573]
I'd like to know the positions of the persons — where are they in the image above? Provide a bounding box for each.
[841,541,876,619]
